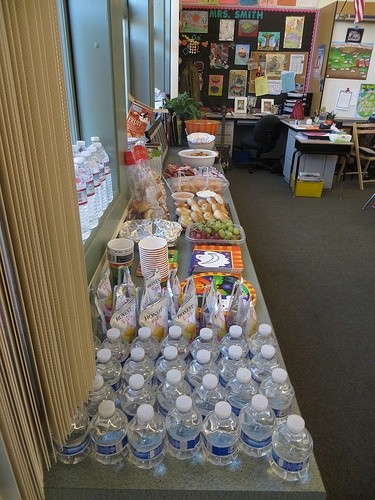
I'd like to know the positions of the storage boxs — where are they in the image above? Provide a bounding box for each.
[290,174,325,197]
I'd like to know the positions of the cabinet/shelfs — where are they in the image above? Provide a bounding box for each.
[215,120,234,159]
[283,128,338,189]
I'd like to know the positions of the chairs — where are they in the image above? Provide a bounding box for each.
[237,115,282,174]
[340,122,375,190]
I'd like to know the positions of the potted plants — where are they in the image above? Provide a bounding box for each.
[326,111,336,125]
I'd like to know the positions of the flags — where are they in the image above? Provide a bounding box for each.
[354,0,364,24]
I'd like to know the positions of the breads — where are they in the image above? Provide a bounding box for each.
[174,193,230,228]
[173,179,221,194]
[133,176,167,220]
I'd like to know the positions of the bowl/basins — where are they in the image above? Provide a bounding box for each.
[184,119,221,136]
[178,149,218,169]
[187,133,216,150]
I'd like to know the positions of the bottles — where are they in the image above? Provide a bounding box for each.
[73,136,114,241]
[52,323,314,481]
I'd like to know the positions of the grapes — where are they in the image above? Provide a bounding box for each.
[189,220,241,241]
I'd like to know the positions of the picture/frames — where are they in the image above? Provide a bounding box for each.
[261,99,274,113]
[235,97,247,113]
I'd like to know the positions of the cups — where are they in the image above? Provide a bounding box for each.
[107,238,134,285]
[138,237,170,291]
[170,192,195,205]
[196,191,216,201]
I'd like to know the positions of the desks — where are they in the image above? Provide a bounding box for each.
[43,147,327,500]
[204,111,333,133]
[288,135,354,200]
